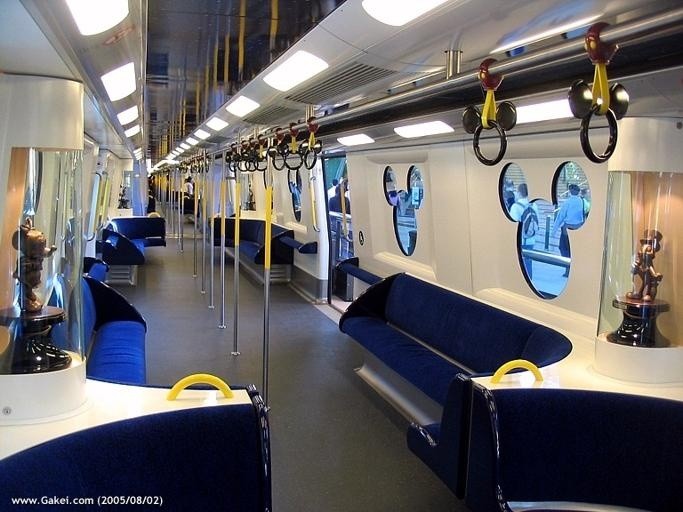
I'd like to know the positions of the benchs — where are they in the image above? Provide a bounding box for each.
[207,216,294,286]
[338,268,574,449]
[147,196,156,212]
[176,192,199,214]
[33,273,259,396]
[97,216,166,288]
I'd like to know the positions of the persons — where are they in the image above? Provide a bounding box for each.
[147,174,195,213]
[291,170,590,282]
